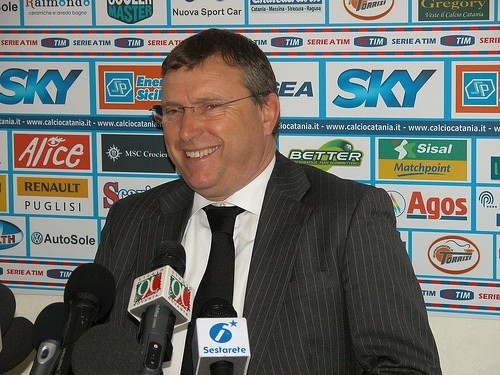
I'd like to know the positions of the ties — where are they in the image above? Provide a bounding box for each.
[179,204,247,375]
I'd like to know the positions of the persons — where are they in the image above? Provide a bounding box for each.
[93,28,442,375]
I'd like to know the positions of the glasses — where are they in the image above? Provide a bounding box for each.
[148,94,255,125]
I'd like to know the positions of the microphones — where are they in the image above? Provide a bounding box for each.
[54,264,115,375]
[29,301,66,375]
[137,238,187,374]
[198,297,238,375]
[70,321,145,375]
